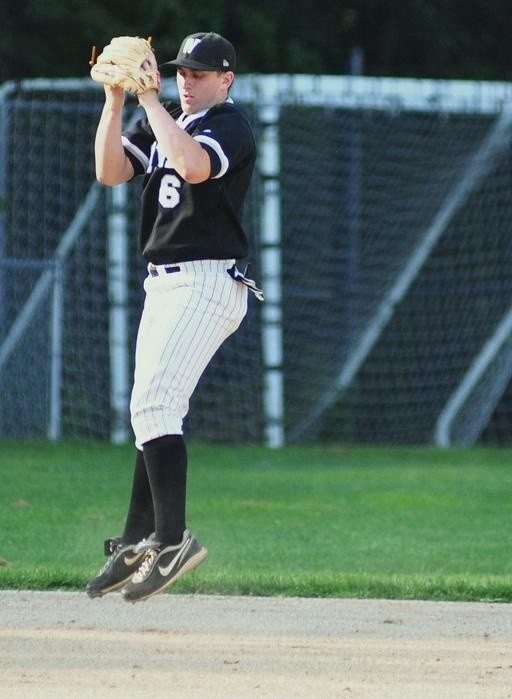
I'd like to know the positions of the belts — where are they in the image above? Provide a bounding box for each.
[149,266,182,279]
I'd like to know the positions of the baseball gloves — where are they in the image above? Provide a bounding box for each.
[90,36,160,93]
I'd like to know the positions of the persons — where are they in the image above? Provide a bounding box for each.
[88,30,258,605]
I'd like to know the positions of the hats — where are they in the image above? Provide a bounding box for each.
[160,32,236,71]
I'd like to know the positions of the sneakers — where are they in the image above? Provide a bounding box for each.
[120,527,207,603]
[86,532,155,600]
